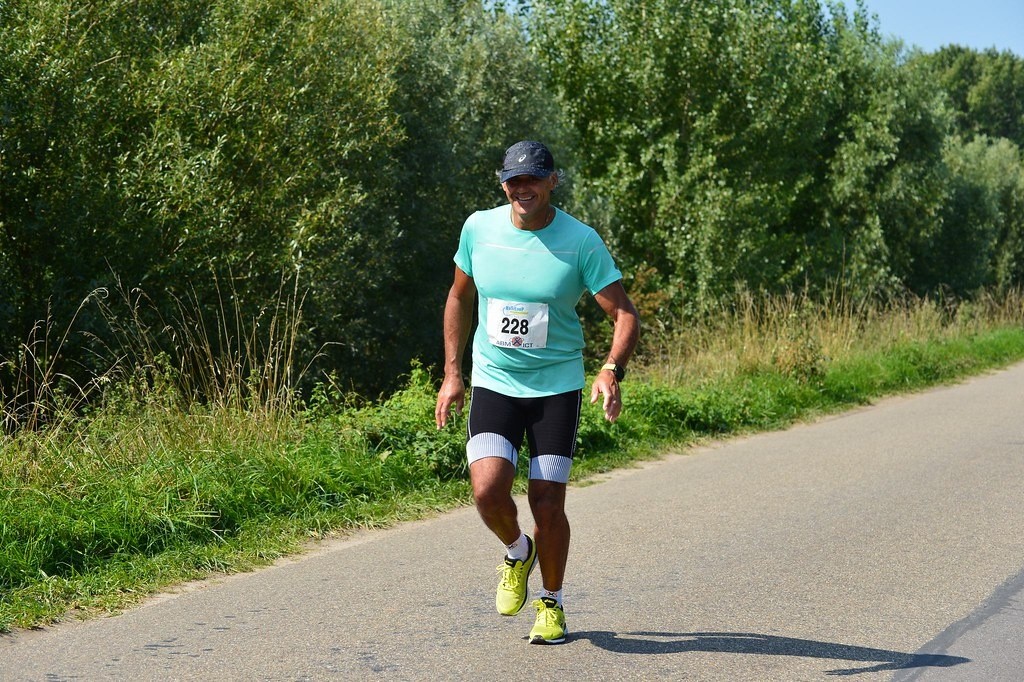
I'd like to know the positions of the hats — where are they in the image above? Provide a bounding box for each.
[500,141,555,184]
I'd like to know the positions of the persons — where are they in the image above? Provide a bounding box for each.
[435,140,639,644]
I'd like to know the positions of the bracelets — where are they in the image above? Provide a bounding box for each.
[601,364,626,382]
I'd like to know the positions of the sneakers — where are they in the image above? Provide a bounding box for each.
[495,533,539,616]
[529,596,568,645]
